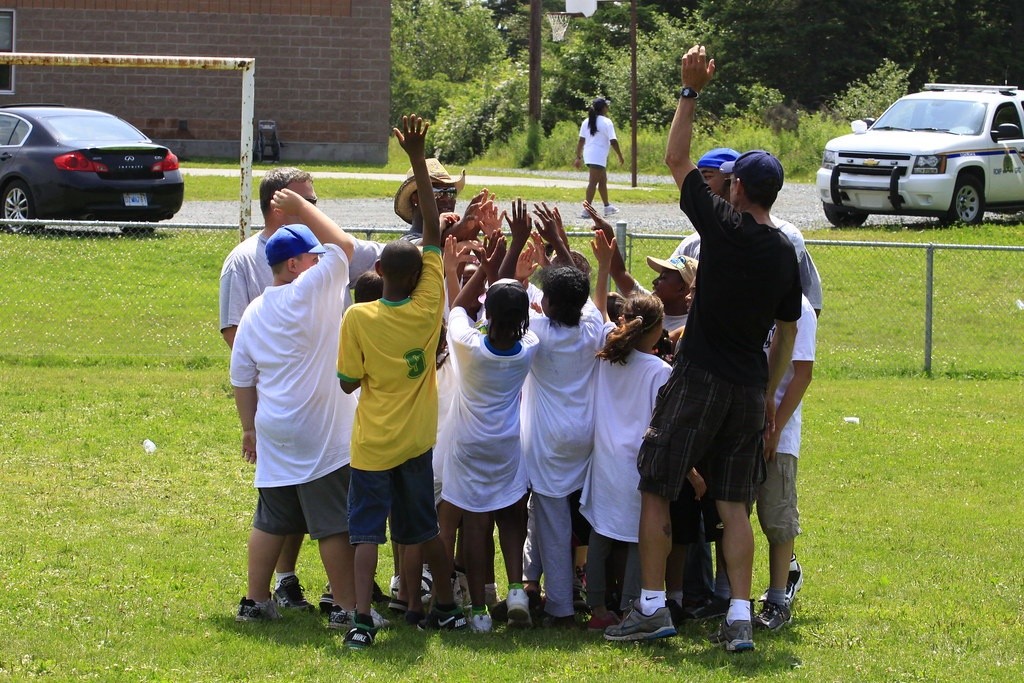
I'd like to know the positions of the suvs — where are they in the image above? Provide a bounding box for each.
[817,85,1024,227]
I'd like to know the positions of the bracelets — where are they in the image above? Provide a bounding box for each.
[577,157,581,159]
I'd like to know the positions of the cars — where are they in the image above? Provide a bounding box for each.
[0,104,184,237]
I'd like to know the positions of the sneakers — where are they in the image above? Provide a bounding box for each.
[272,575,315,612]
[760,562,803,606]
[235,591,284,622]
[684,592,731,622]
[327,603,356,629]
[665,597,688,629]
[348,567,632,633]
[752,600,793,632]
[581,208,591,218]
[707,609,755,652]
[318,593,334,615]
[603,204,620,216]
[342,622,380,650]
[603,597,677,641]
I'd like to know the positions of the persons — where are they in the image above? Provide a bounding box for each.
[575,98,624,216]
[220,113,699,650]
[605,45,801,652]
[750,291,817,631]
[669,149,823,605]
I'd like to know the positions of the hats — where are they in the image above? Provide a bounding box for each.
[394,158,466,225]
[264,223,326,266]
[647,254,698,286]
[719,149,785,192]
[697,147,742,169]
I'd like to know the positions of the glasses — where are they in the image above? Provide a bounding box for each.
[307,198,318,205]
[412,187,457,205]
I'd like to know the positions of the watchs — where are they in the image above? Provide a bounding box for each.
[680,87,699,99]
